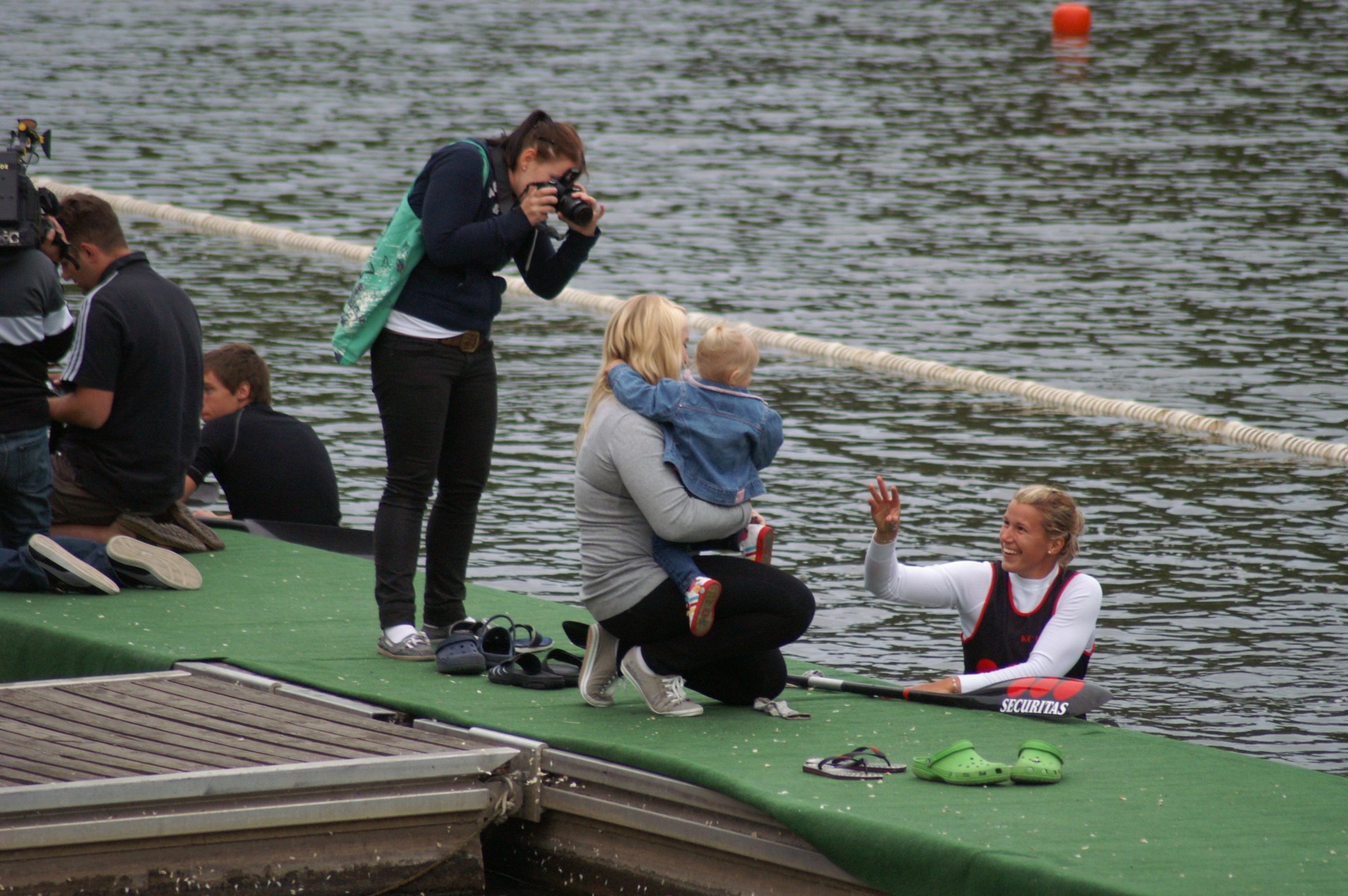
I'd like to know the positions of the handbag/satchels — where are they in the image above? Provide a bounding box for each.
[330,140,491,365]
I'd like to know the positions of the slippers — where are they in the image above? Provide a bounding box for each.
[541,648,583,687]
[912,741,1012,785]
[435,633,486,674]
[489,653,564,690]
[508,623,553,653]
[1010,739,1065,782]
[802,746,907,780]
[446,618,499,637]
[478,614,516,667]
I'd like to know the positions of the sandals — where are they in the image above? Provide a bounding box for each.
[168,502,226,551]
[112,514,207,553]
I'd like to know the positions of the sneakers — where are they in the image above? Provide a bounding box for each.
[620,646,703,718]
[578,624,619,708]
[686,576,722,634]
[28,534,120,596]
[422,617,476,643]
[739,524,775,565]
[105,535,202,591]
[377,630,436,662]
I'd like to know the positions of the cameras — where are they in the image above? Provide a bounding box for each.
[536,168,594,227]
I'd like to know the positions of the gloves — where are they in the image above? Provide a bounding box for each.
[754,697,812,720]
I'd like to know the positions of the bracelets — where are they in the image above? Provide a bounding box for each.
[952,676,957,690]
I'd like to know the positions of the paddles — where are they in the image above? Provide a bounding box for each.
[562,618,1114,719]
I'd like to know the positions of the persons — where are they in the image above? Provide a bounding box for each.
[368,110,604,662]
[0,193,225,595]
[574,294,815,717]
[864,476,1103,721]
[179,344,342,528]
